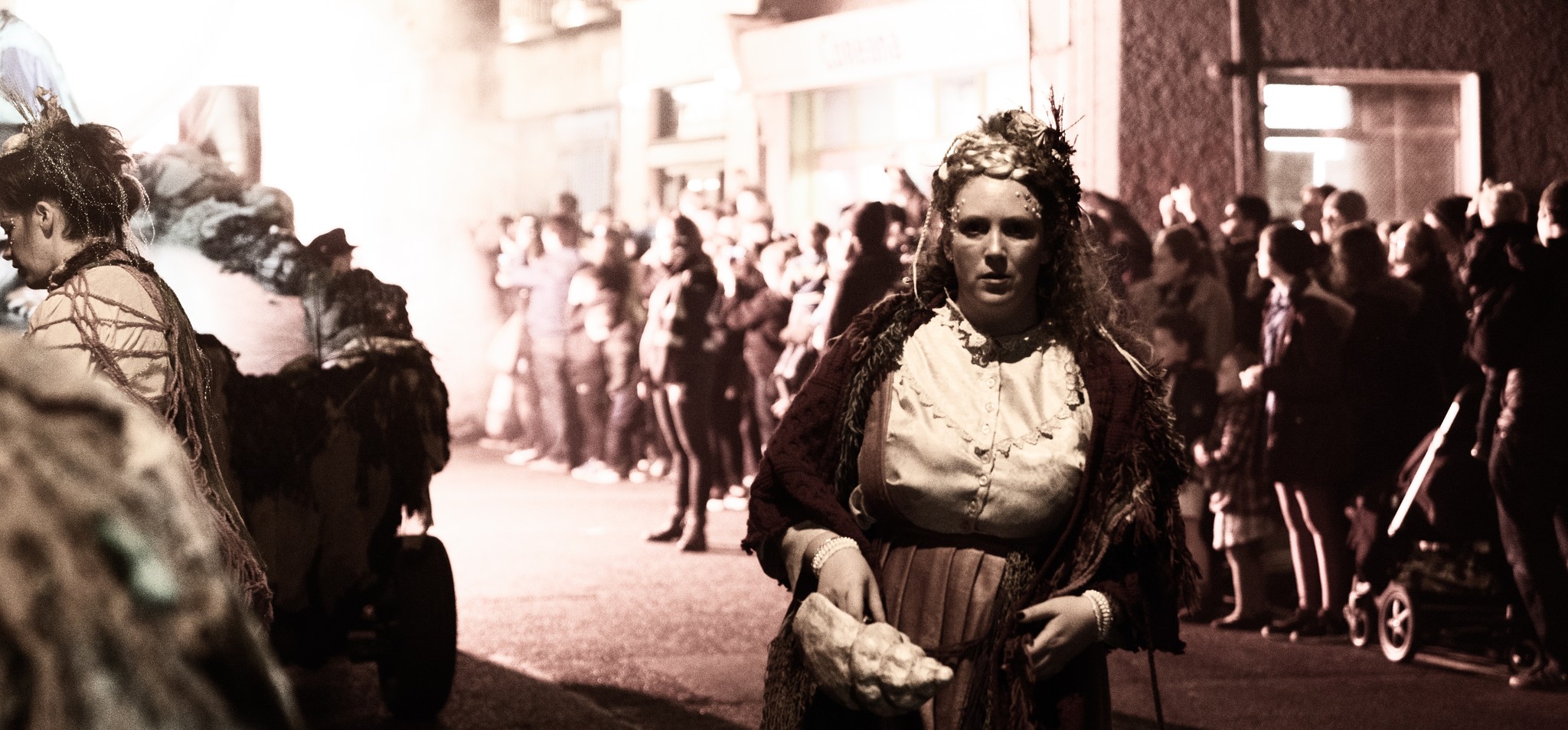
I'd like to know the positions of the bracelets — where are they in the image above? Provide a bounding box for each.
[812,537,859,574]
[1083,593,1102,635]
[1085,590,1111,643]
[815,535,844,557]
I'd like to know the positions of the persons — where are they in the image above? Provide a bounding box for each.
[0,0,1568,730]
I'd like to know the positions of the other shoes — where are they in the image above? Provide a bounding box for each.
[1213,610,1260,629]
[1261,603,1317,643]
[1182,604,1217,622]
[650,529,682,542]
[1509,657,1568,689]
[481,434,627,483]
[1289,612,1345,644]
[682,531,706,552]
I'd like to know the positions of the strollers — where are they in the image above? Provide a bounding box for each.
[1344,369,1533,670]
